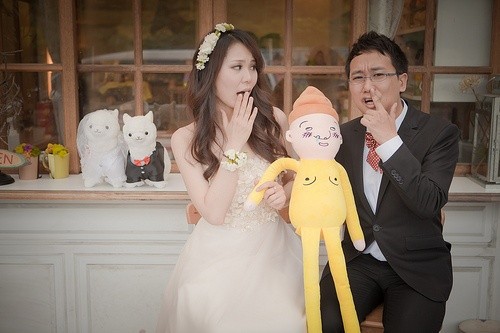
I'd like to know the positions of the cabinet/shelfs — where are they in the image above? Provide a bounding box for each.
[0,175,500,333]
[394,0,493,104]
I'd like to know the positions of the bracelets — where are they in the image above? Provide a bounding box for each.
[223,151,248,172]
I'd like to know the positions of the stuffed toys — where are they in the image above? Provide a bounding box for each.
[79,109,129,187]
[121,111,171,188]
[244,86,365,333]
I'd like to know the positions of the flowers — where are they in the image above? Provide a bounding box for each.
[195,22,235,71]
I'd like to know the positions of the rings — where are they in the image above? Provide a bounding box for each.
[273,186,277,193]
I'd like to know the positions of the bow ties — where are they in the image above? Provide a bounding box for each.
[365,132,384,174]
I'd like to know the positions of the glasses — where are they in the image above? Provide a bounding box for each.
[348,71,401,84]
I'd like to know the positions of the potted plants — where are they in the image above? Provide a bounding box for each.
[14,143,70,181]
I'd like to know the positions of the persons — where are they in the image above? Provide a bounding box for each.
[158,23,307,333]
[319,31,460,333]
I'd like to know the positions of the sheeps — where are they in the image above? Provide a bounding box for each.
[123,110,171,188]
[84,109,127,188]
